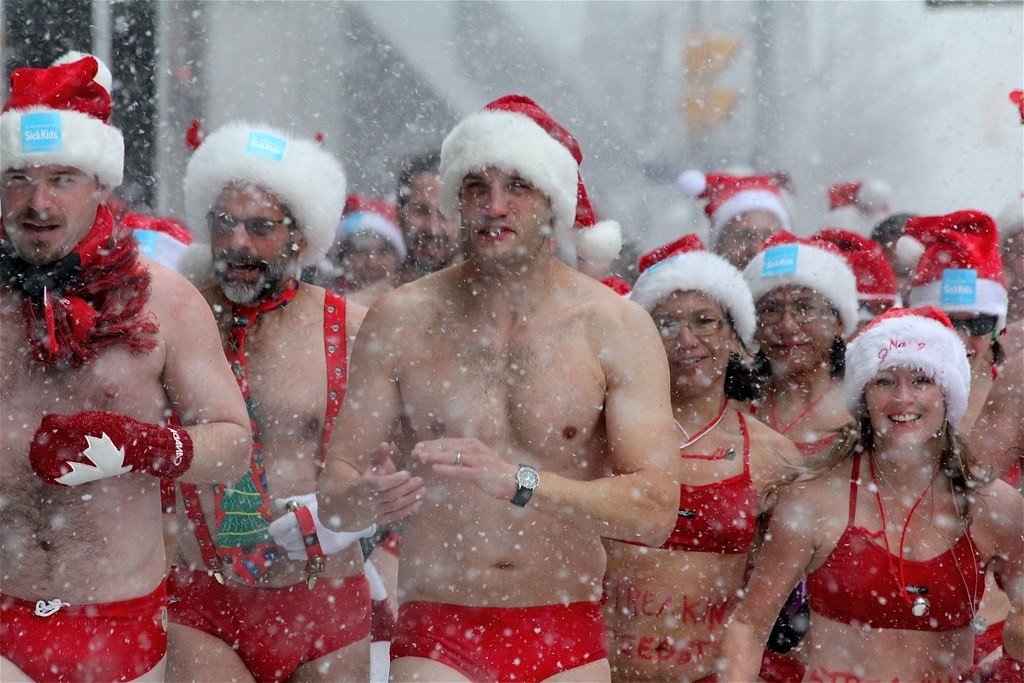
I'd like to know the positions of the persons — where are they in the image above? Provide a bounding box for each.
[723,312,1024,683]
[0,48,253,681]
[313,91,682,683]
[169,121,380,683]
[737,230,859,459]
[605,252,805,683]
[337,130,1024,375]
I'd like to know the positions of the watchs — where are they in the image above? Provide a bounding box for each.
[506,463,542,510]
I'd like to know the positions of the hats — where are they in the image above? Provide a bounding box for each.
[120,212,213,283]
[892,208,1009,328]
[739,227,860,341]
[2,48,125,189]
[337,193,407,265]
[765,225,898,322]
[180,118,351,265]
[842,307,972,427]
[676,170,792,255]
[614,232,757,353]
[820,180,896,244]
[991,190,1024,256]
[437,95,625,268]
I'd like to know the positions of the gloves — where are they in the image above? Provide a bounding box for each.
[267,492,376,561]
[29,410,195,489]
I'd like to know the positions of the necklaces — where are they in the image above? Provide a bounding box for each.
[860,458,947,620]
[762,377,838,434]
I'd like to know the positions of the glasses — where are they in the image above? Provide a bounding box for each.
[652,314,729,342]
[752,299,827,325]
[202,210,292,239]
[933,314,996,337]
